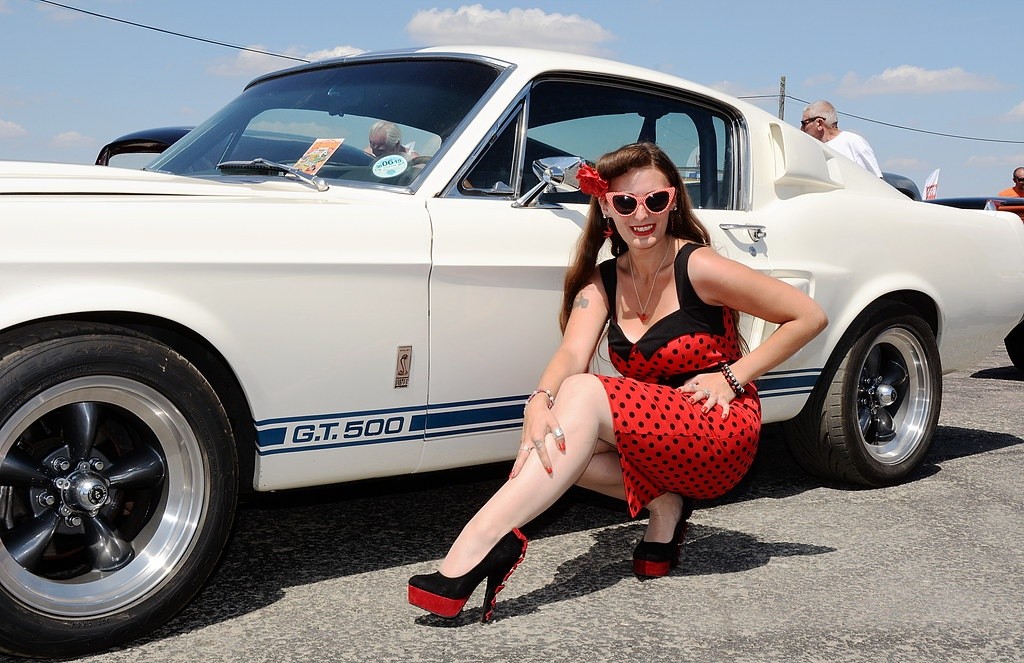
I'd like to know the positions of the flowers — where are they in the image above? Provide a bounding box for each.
[576,164,609,199]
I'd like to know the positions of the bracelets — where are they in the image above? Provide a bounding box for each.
[523,387,555,415]
[718,360,749,398]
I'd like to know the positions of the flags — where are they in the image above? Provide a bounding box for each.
[922,167,940,200]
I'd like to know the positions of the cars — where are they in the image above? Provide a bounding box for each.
[93,124,1024,221]
[0,47,1024,662]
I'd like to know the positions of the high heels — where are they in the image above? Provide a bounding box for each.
[408,527,528,622]
[633,496,693,577]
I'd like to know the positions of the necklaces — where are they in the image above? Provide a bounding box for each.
[627,234,672,323]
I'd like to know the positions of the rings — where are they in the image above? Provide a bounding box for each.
[689,383,698,392]
[552,428,565,440]
[520,446,531,453]
[702,388,710,398]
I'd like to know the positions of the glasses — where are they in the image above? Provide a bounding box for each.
[606,187,676,217]
[801,117,826,126]
[1014,176,1024,181]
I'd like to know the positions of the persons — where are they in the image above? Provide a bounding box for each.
[368,120,425,169]
[800,99,883,180]
[997,167,1024,224]
[407,142,829,622]
